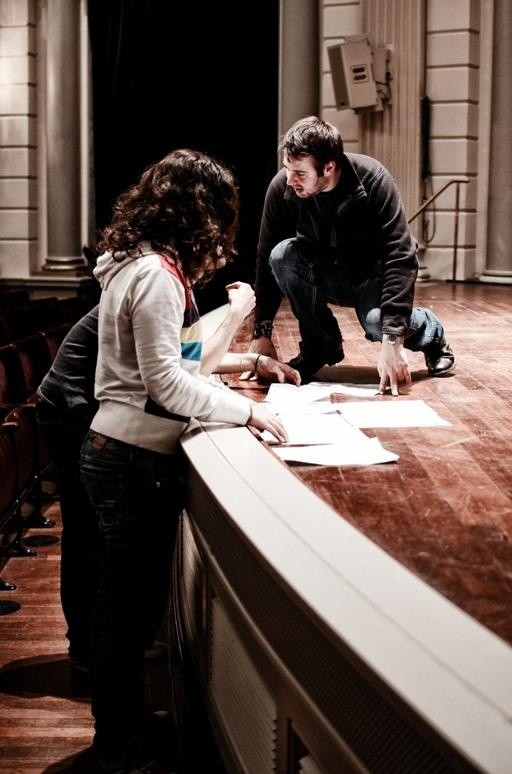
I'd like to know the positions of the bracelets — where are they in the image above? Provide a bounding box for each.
[253,321,272,339]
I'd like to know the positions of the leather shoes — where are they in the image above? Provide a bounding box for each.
[422,335,457,376]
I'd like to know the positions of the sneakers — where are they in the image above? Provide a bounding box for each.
[90,706,175,772]
[283,336,346,380]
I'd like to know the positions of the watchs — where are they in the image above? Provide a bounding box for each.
[382,334,405,344]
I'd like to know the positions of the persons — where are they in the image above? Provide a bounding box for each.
[38,150,299,774]
[238,116,456,396]
